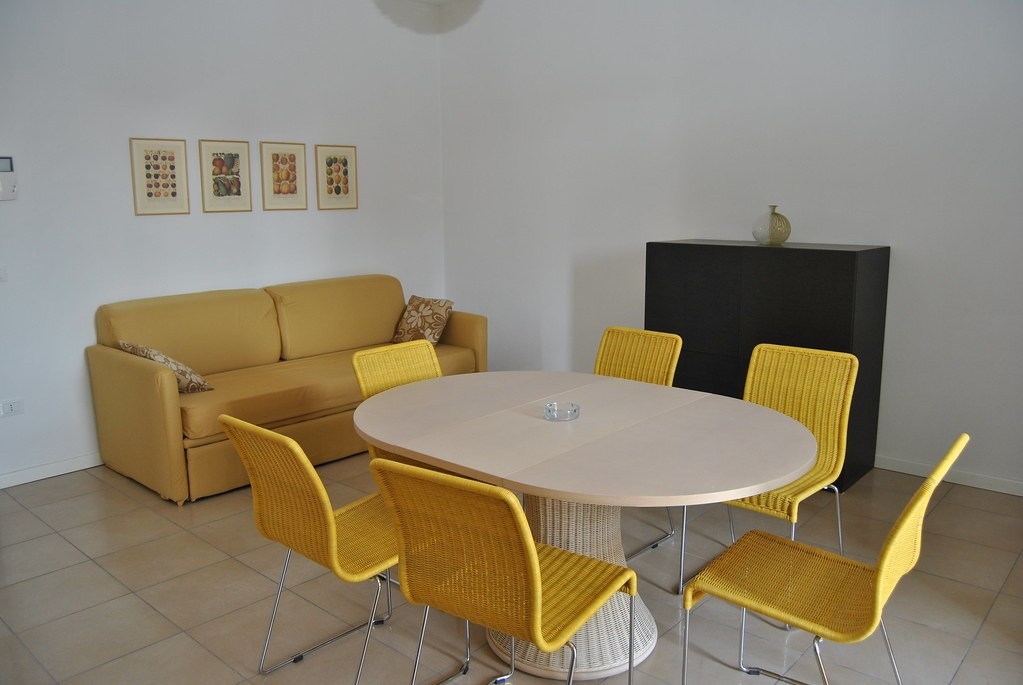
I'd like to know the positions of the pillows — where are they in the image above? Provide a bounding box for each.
[392,293,455,347]
[117,337,214,392]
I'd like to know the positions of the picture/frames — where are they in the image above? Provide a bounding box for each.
[259,141,308,211]
[198,139,253,211]
[129,138,190,216]
[315,144,358,210]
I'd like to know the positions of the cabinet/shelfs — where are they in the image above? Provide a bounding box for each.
[642,239,890,495]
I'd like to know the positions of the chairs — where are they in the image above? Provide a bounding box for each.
[370,457,638,685]
[216,413,400,685]
[677,342,858,595]
[352,339,475,479]
[681,434,970,685]
[594,327,683,560]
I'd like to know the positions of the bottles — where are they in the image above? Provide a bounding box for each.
[751,203,791,245]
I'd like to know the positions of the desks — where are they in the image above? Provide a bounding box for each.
[352,368,820,683]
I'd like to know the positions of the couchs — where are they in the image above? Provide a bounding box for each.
[86,273,488,508]
